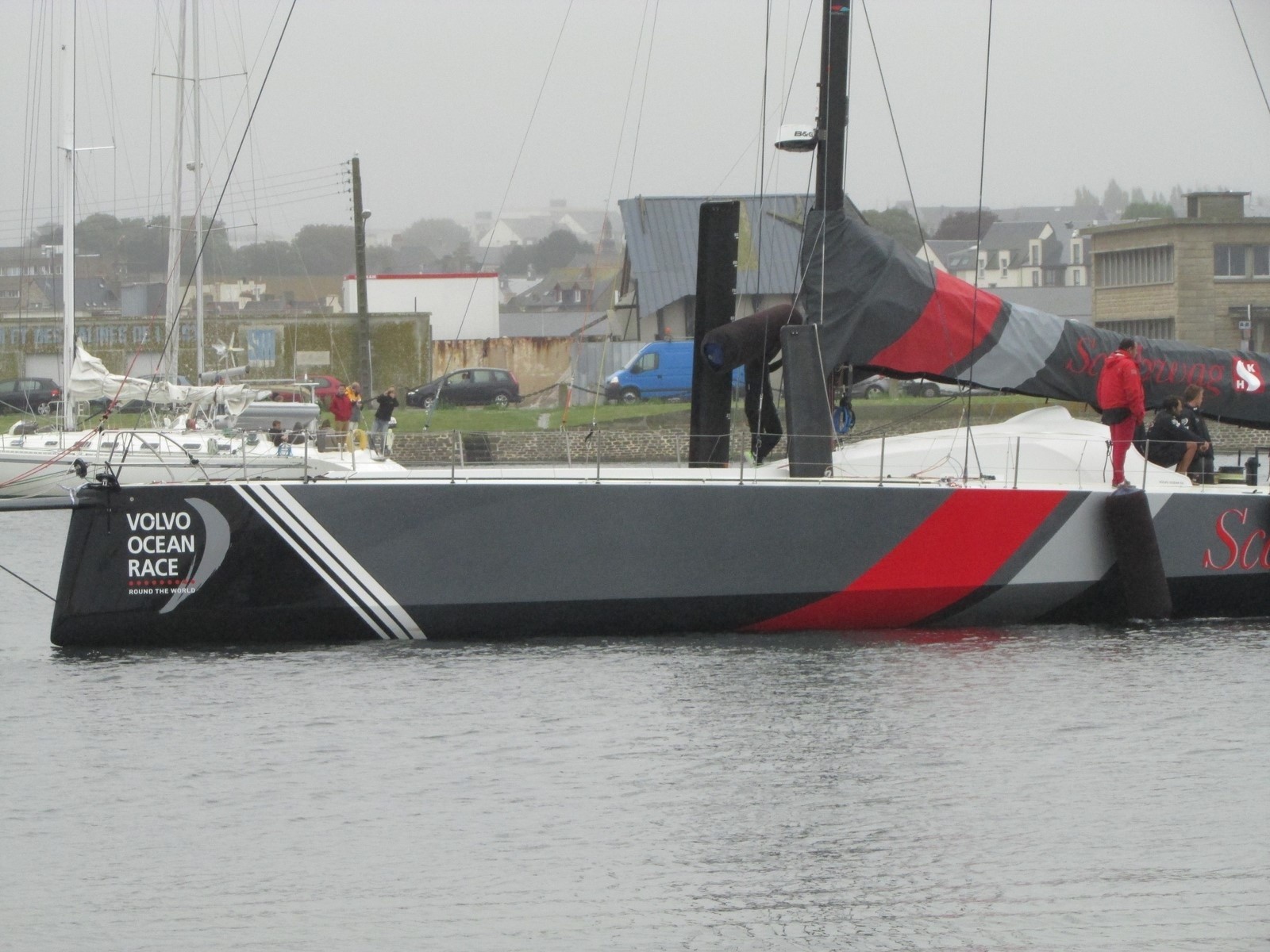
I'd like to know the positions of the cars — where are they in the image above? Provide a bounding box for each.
[897,377,1004,398]
[405,367,523,410]
[0,376,62,417]
[269,375,346,403]
[110,373,194,413]
[832,372,892,400]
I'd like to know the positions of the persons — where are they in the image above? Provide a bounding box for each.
[214,375,226,415]
[330,384,353,452]
[48,390,62,411]
[1179,384,1215,485]
[369,387,399,453]
[1146,396,1210,483]
[1097,338,1145,488]
[288,422,313,442]
[316,420,338,448]
[346,382,364,446]
[268,421,287,446]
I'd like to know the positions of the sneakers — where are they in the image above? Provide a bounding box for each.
[1112,481,1136,490]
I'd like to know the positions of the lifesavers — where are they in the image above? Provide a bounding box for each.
[386,428,392,450]
[347,428,368,453]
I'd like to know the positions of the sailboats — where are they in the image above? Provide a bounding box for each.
[49,0,1270,655]
[0,0,411,515]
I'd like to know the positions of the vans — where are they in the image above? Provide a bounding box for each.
[602,339,747,407]
